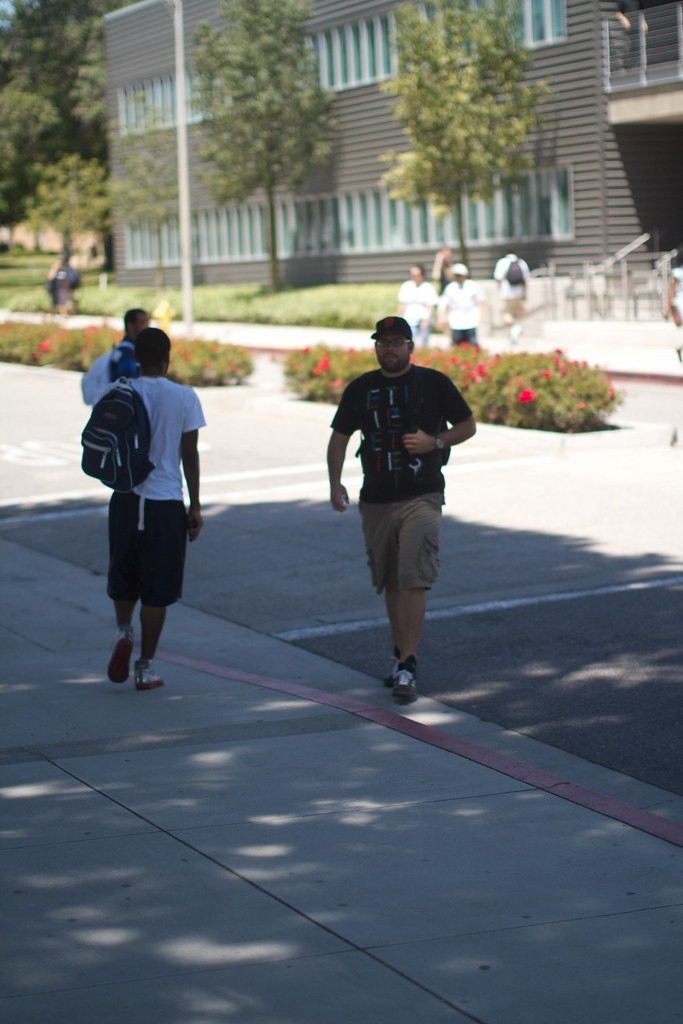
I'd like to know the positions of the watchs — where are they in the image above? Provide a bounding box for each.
[434,434,445,450]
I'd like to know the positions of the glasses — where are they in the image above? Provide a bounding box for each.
[376,337,411,346]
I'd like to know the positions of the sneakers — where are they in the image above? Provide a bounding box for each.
[134,659,162,689]
[384,647,419,700]
[106,625,131,684]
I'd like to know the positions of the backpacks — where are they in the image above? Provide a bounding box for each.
[499,256,525,286]
[80,341,136,410]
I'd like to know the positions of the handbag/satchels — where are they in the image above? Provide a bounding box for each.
[79,378,156,494]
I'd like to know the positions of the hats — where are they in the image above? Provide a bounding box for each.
[371,317,413,339]
[452,262,469,277]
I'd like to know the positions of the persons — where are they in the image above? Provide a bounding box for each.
[396,261,438,346]
[46,247,79,317]
[494,248,530,351]
[432,247,452,295]
[437,263,488,353]
[327,316,476,697]
[109,309,149,383]
[106,328,206,690]
[669,268,683,449]
